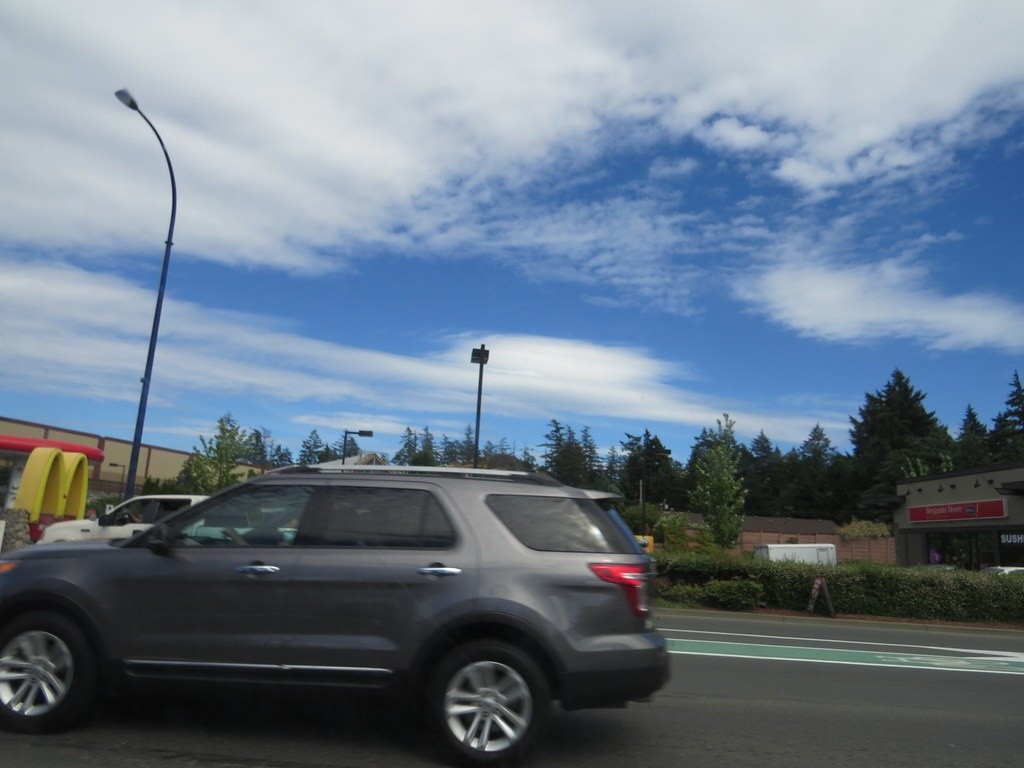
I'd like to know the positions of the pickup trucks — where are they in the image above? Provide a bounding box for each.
[36,494,297,544]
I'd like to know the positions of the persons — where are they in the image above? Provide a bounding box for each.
[127,509,144,522]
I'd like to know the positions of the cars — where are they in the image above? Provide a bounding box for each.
[979,567,1023,577]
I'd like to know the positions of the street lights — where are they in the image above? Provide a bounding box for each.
[472,343,489,471]
[116,87,177,503]
[340,431,373,472]
[108,462,124,500]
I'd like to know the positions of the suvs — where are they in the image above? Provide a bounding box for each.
[1,460,677,768]
